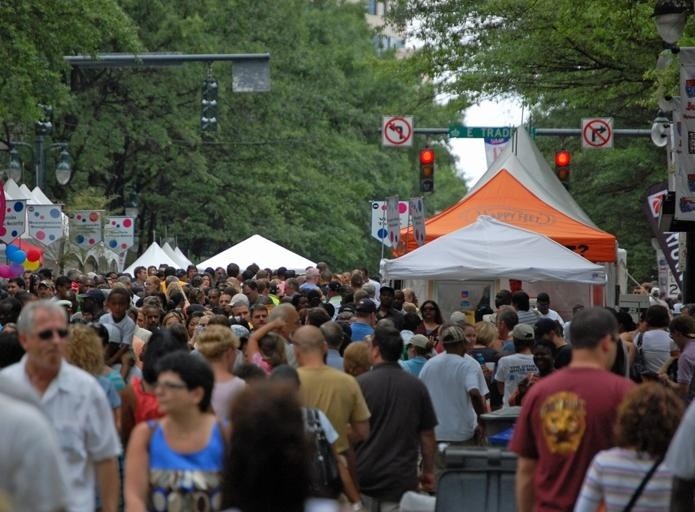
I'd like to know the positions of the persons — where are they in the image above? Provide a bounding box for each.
[510,304,640,511]
[1,391,76,512]
[123,348,234,512]
[0,300,122,512]
[221,383,312,511]
[349,328,438,511]
[260,363,368,512]
[575,378,685,512]
[281,326,371,455]
[418,326,486,451]
[1,260,695,441]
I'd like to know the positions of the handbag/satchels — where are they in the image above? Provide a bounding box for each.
[399,490,436,512]
[305,411,341,497]
[629,348,646,382]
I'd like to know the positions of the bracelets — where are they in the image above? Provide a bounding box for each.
[352,499,365,511]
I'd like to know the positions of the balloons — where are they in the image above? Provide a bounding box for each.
[0,242,43,279]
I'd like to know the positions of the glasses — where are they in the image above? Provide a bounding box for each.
[38,330,68,340]
[151,382,186,389]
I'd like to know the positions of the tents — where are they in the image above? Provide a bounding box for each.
[121,240,193,280]
[196,234,317,276]
[379,217,607,308]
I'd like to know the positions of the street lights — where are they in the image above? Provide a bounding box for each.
[8,141,71,191]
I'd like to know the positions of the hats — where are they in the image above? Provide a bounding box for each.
[79,287,105,299]
[355,298,376,311]
[39,280,50,287]
[440,326,469,343]
[507,324,535,340]
[380,286,393,293]
[408,334,432,350]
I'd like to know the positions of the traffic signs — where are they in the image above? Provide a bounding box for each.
[449,127,517,138]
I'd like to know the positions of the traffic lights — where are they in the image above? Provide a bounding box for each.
[421,150,433,192]
[555,151,570,193]
